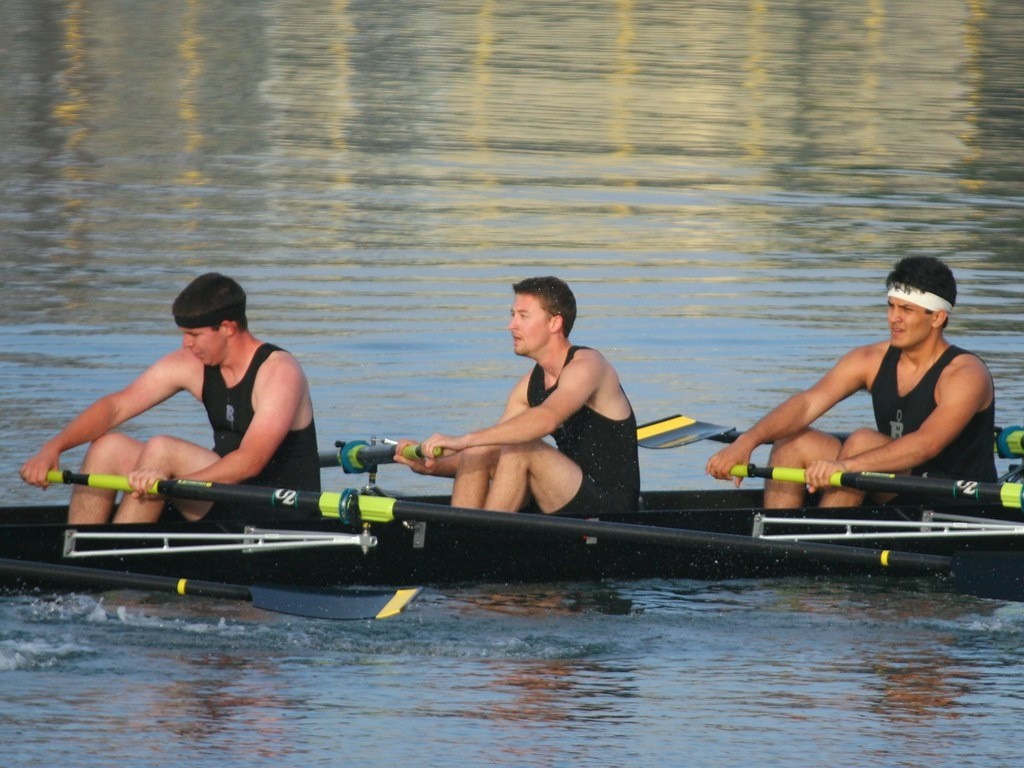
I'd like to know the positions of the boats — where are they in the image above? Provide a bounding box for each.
[1,426,1024,584]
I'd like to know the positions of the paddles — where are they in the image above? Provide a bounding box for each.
[18,464,1022,594]
[630,412,1023,458]
[713,464,1024,515]
[1,555,425,619]
[313,444,447,470]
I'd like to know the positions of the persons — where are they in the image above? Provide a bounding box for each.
[392,276,640,515]
[705,254,1000,507]
[18,272,323,525]
